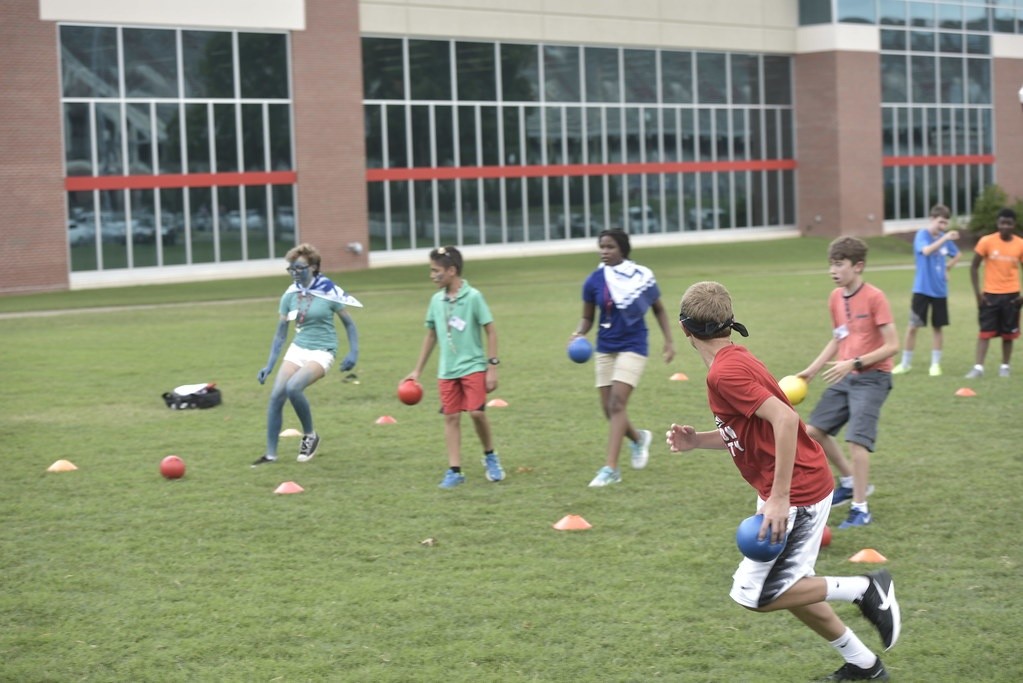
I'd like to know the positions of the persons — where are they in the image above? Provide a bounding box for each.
[398,245,505,487]
[665,280,901,683]
[793,236,899,532]
[567,226,675,489]
[966,208,1023,378]
[249,244,358,466]
[890,204,961,376]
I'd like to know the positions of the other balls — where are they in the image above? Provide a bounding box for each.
[736,513,786,563]
[568,338,593,364]
[778,374,808,407]
[820,524,832,547]
[397,380,424,406]
[160,455,185,478]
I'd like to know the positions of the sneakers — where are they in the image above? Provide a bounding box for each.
[297,431,320,461]
[628,429,653,470]
[481,450,506,482]
[249,454,280,468]
[831,476,875,507]
[852,572,901,653]
[438,469,465,489]
[587,466,622,487]
[825,654,890,682]
[837,505,873,531]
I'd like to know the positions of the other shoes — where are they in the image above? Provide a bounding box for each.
[964,368,982,379]
[929,363,942,377]
[891,363,913,375]
[1000,368,1010,379]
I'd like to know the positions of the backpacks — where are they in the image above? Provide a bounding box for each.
[162,383,223,410]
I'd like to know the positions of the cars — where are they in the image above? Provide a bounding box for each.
[66,207,297,248]
[560,202,730,236]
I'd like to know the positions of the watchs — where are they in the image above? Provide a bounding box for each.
[487,358,499,364]
[852,357,862,372]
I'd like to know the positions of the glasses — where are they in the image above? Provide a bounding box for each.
[437,246,459,270]
[286,264,313,275]
